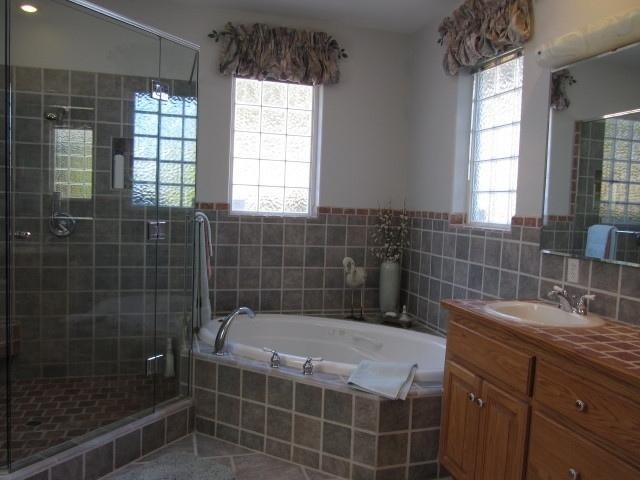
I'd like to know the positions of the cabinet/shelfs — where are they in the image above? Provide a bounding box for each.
[436,319,536,480]
[525,355,640,479]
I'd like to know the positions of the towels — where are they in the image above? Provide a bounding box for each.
[585,223,618,261]
[346,360,419,401]
[192,211,213,329]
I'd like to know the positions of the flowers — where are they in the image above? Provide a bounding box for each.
[368,200,411,265]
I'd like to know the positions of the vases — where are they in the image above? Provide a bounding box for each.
[379,262,401,313]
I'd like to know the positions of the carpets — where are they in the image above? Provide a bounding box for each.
[115,452,233,480]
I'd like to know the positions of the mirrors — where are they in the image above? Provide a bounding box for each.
[539,40,640,269]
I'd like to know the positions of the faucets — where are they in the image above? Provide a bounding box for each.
[546,283,573,314]
[214,306,256,356]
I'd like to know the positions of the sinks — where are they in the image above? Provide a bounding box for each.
[483,300,606,329]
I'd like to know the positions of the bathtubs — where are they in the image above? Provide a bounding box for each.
[190,311,450,382]
